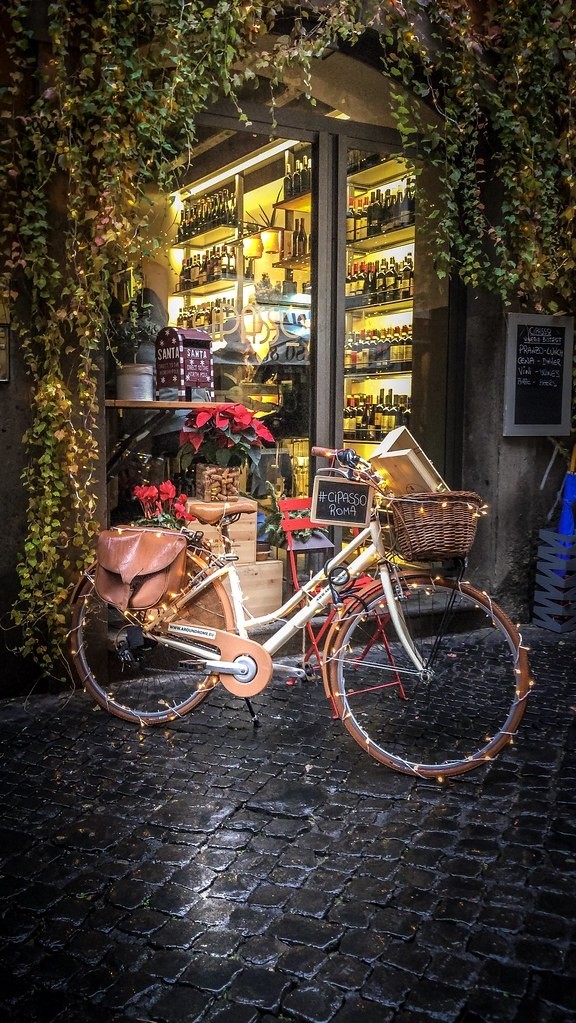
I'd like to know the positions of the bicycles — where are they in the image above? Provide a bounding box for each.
[65,445,529,780]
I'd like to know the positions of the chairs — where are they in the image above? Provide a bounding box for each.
[278,498,409,719]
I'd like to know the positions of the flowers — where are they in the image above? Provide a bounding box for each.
[134,480,196,531]
[253,273,281,301]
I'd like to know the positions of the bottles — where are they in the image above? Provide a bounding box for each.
[345,252,413,308]
[298,218,307,257]
[284,252,292,262]
[292,219,300,256]
[178,189,236,242]
[179,242,255,293]
[244,297,253,313]
[284,155,311,199]
[344,324,412,374]
[347,148,378,175]
[346,178,415,242]
[176,297,234,328]
[342,389,411,441]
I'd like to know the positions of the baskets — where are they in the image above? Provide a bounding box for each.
[392,490,487,562]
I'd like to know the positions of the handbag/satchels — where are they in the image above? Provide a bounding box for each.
[94,524,187,609]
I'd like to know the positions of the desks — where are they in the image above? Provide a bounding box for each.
[105,399,240,483]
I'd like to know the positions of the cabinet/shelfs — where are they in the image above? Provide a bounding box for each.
[169,110,416,461]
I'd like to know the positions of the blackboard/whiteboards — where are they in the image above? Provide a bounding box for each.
[503,311,574,436]
[310,474,374,529]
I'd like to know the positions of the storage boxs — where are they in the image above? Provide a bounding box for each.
[369,425,451,497]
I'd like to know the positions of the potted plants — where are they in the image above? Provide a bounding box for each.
[108,309,163,402]
[229,185,284,259]
[180,404,275,502]
